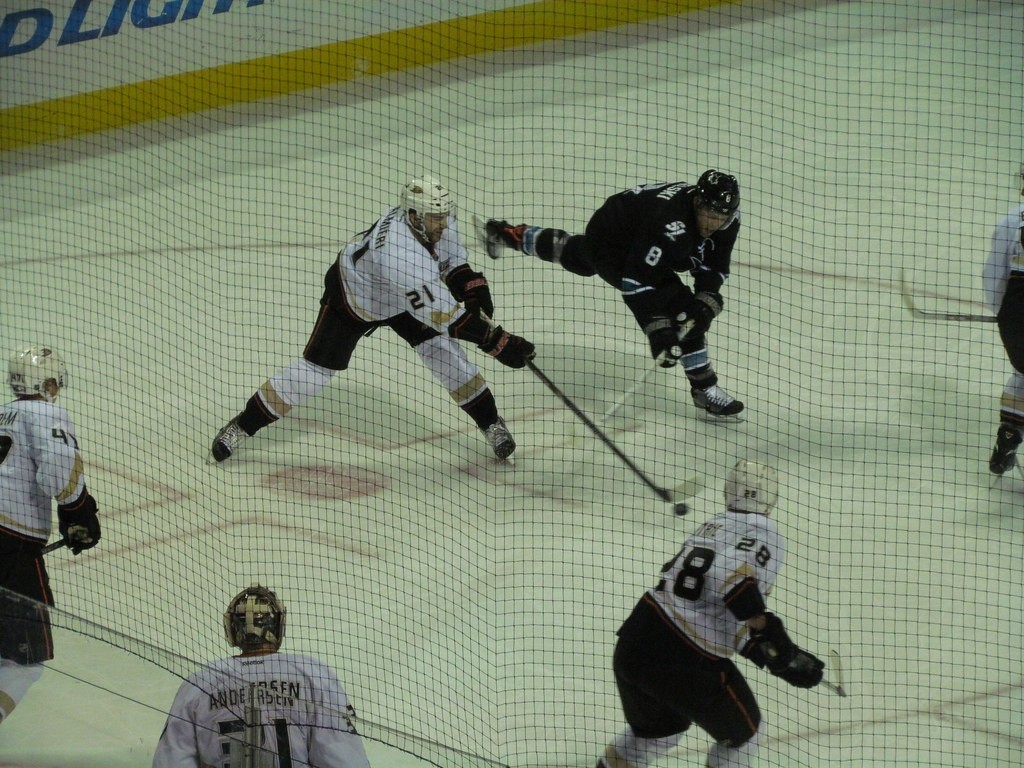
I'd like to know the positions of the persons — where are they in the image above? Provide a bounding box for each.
[207,174,536,465]
[148,584,372,768]
[0,345,101,724]
[473,170,746,422]
[594,460,825,768]
[981,162,1024,473]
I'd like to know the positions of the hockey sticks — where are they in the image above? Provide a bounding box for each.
[768,648,846,697]
[41,530,88,554]
[527,358,706,503]
[898,269,998,323]
[594,319,694,424]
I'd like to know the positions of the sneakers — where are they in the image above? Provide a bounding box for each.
[204,411,250,466]
[989,424,1023,474]
[690,382,744,425]
[473,214,504,260]
[479,416,517,466]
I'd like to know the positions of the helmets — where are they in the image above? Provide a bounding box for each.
[401,175,455,224]
[7,342,68,395]
[693,168,741,218]
[223,587,293,646]
[724,457,779,515]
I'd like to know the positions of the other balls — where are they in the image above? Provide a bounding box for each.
[674,504,688,516]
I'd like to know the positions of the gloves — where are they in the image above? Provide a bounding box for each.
[57,489,102,554]
[738,612,825,689]
[677,290,725,341]
[446,263,493,321]
[448,309,534,369]
[642,311,681,367]
